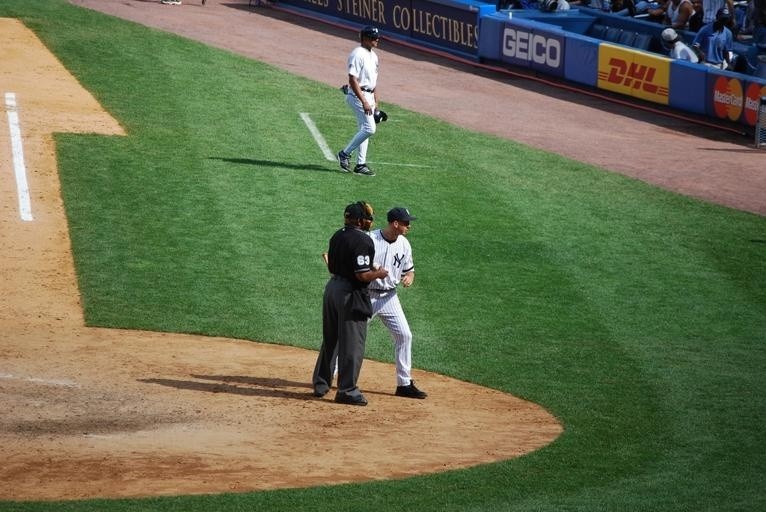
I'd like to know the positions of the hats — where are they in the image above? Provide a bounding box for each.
[661,28,681,43]
[359,26,382,40]
[387,207,417,221]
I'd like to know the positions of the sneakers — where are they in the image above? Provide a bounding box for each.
[336,151,351,173]
[353,165,375,176]
[395,379,427,399]
[336,391,368,405]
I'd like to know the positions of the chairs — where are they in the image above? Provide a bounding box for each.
[590,24,654,51]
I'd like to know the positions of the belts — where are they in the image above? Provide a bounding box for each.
[361,87,376,93]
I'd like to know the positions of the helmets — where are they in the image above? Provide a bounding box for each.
[344,201,374,231]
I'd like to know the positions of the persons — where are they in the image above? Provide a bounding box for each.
[314,198,388,406]
[333,208,428,400]
[543,0,753,72]
[336,26,380,177]
